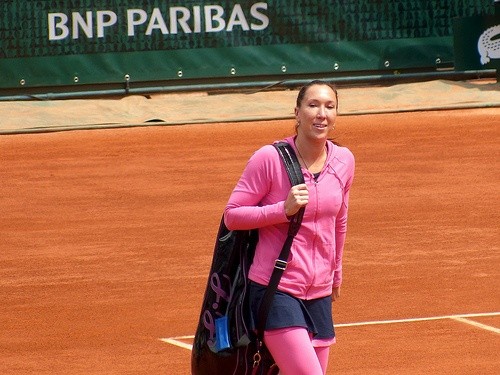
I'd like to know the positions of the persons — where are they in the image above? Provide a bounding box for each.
[223,80,355,375]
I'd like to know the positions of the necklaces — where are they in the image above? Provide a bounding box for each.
[294,139,308,170]
